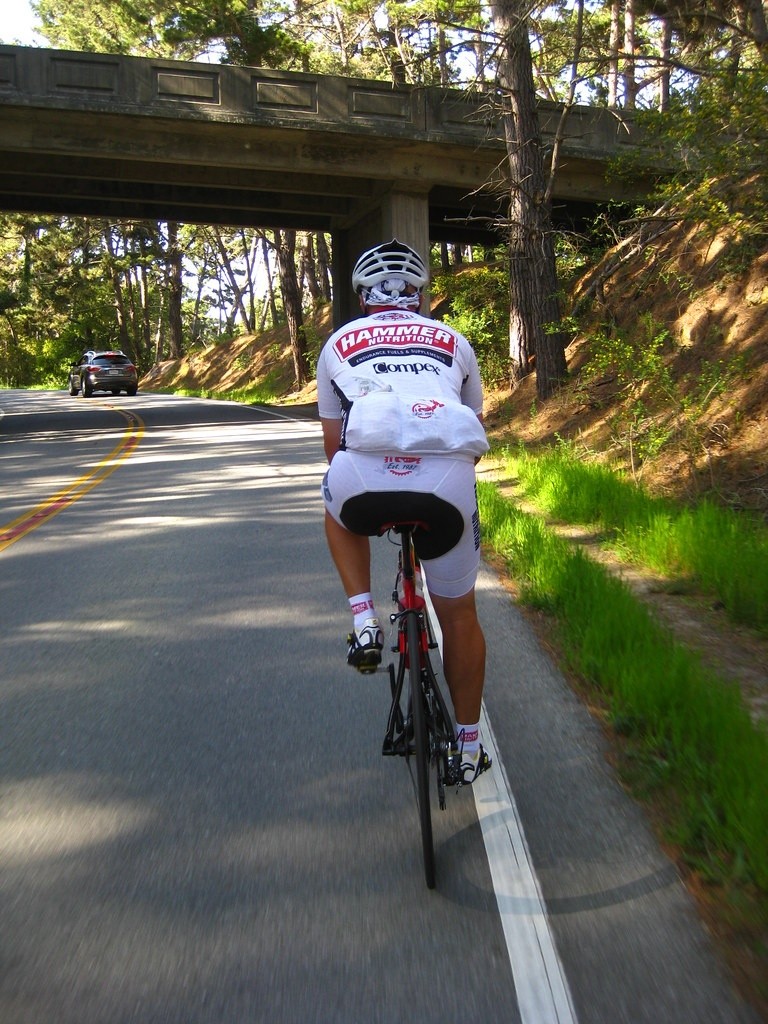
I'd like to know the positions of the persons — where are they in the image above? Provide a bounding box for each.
[317,243,494,787]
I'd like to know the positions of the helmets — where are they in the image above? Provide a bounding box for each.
[352,237,428,293]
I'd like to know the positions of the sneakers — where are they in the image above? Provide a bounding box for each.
[346,618,384,666]
[448,743,492,785]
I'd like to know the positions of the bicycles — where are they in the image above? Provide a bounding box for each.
[347,512,488,889]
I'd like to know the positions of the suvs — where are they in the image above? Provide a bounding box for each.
[68,350,139,398]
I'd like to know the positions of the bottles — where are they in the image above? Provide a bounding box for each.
[398,567,429,669]
